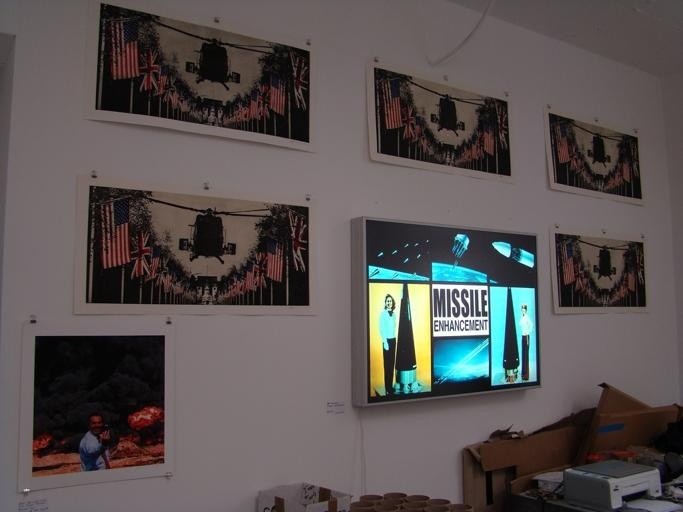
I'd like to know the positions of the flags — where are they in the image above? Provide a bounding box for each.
[105,14,308,131]
[91,188,308,305]
[375,73,509,170]
[555,237,645,309]
[550,117,641,193]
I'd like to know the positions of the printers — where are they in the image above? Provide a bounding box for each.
[563,460,664,512]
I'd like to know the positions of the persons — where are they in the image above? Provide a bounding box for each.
[517,304,533,380]
[78,412,114,471]
[377,293,396,394]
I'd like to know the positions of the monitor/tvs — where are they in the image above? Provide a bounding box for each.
[349,214,541,411]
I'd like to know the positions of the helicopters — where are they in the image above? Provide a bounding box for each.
[149,15,278,91]
[402,77,490,136]
[138,197,281,265]
[569,123,625,169]
[570,239,631,281]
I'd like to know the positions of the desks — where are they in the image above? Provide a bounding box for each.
[515,477,683,512]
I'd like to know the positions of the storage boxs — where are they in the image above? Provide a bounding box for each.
[256,481,351,512]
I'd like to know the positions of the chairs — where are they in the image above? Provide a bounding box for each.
[504,465,569,495]
